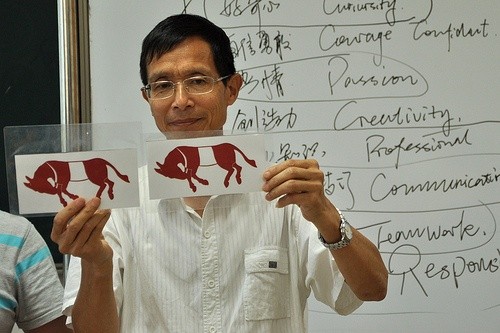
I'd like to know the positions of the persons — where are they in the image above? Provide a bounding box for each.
[51,13,389,333]
[1,208,74,333]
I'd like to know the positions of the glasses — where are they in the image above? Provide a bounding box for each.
[142,74,233,100]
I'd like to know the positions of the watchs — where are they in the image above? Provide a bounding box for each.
[318,207,352,248]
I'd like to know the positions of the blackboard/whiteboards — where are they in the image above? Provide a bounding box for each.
[76,0,500,333]
[0,0,79,273]
